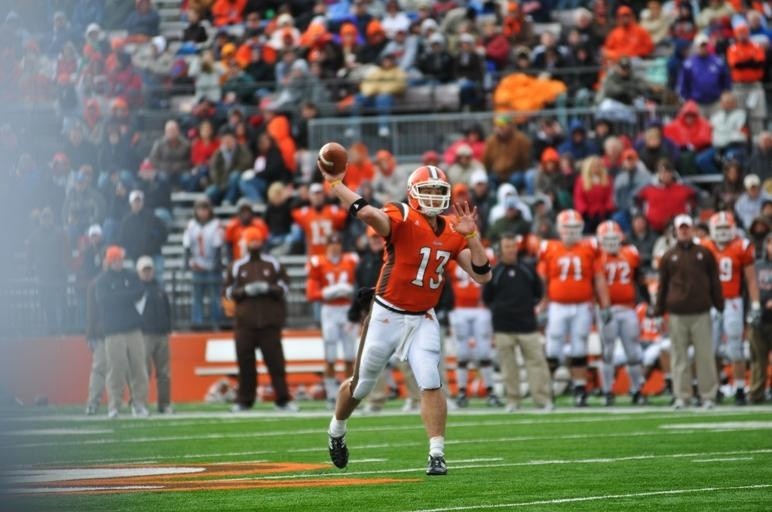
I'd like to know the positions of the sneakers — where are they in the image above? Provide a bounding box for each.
[86,405,174,417]
[228,403,247,411]
[426,452,448,475]
[327,393,471,410]
[274,404,300,412]
[488,384,747,413]
[328,428,348,468]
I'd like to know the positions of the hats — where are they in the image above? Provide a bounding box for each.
[108,247,123,260]
[137,255,155,270]
[87,224,102,236]
[743,173,759,189]
[674,214,692,228]
[129,190,143,202]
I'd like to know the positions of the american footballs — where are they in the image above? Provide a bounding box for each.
[318,141,347,175]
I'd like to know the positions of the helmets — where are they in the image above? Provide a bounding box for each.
[556,210,584,244]
[710,212,736,242]
[599,220,622,254]
[405,166,451,218]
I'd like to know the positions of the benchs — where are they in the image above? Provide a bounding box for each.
[190,333,631,400]
[11,0,311,305]
